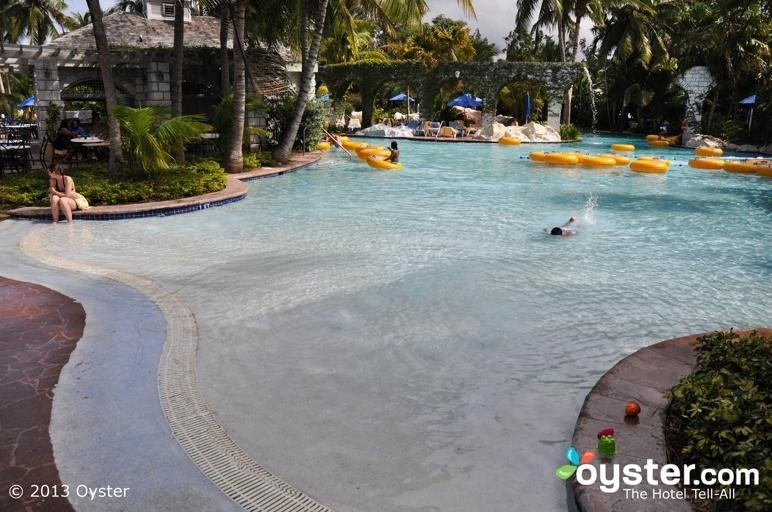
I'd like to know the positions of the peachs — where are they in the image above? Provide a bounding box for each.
[625,401,640,415]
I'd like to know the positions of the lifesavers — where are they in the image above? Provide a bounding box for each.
[530,143,669,173]
[687,145,772,177]
[646,133,682,147]
[499,136,521,145]
[315,132,402,170]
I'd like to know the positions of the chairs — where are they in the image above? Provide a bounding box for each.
[0,119,111,176]
[348,110,480,139]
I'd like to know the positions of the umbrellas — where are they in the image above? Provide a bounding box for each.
[15,94,38,120]
[738,94,757,134]
[388,92,415,109]
[523,89,532,124]
[444,92,483,126]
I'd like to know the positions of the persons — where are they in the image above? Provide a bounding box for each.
[541,213,591,238]
[424,117,435,136]
[52,117,81,166]
[322,126,341,151]
[71,118,90,159]
[384,110,412,128]
[383,141,400,163]
[511,117,519,126]
[464,114,470,126]
[46,163,89,226]
